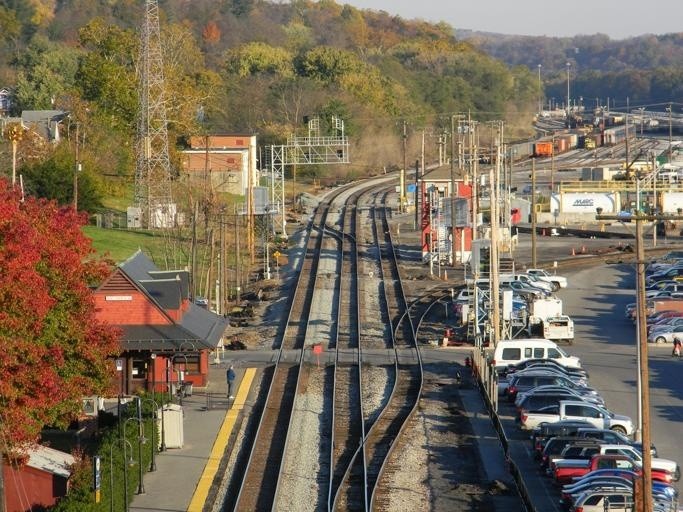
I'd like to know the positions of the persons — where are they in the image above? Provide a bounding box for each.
[226,364,235,399]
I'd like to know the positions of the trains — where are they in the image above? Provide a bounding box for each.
[534,107,683,158]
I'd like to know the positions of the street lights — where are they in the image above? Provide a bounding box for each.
[538,63,542,117]
[108,341,201,510]
[566,61,572,129]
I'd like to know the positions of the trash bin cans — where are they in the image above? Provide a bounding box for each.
[185,382,192,396]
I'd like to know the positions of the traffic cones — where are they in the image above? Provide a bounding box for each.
[570,247,577,257]
